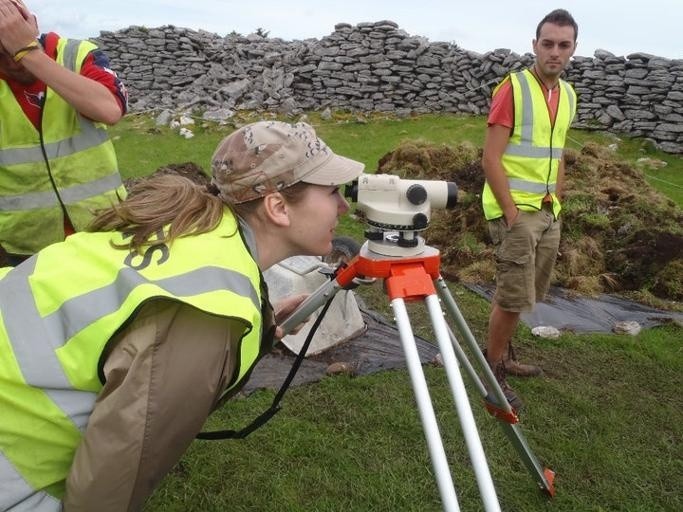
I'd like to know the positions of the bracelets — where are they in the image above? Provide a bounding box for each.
[10,40,40,65]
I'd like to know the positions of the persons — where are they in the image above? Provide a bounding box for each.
[478,8,580,410]
[0,0,130,269]
[0,120,367,511]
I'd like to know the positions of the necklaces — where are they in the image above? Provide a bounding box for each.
[532,62,559,104]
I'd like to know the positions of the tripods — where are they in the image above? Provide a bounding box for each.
[271,237,558,512]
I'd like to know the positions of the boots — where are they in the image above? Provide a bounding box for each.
[506,342,542,376]
[478,349,523,409]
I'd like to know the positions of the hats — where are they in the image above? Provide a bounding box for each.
[210,119,366,206]
